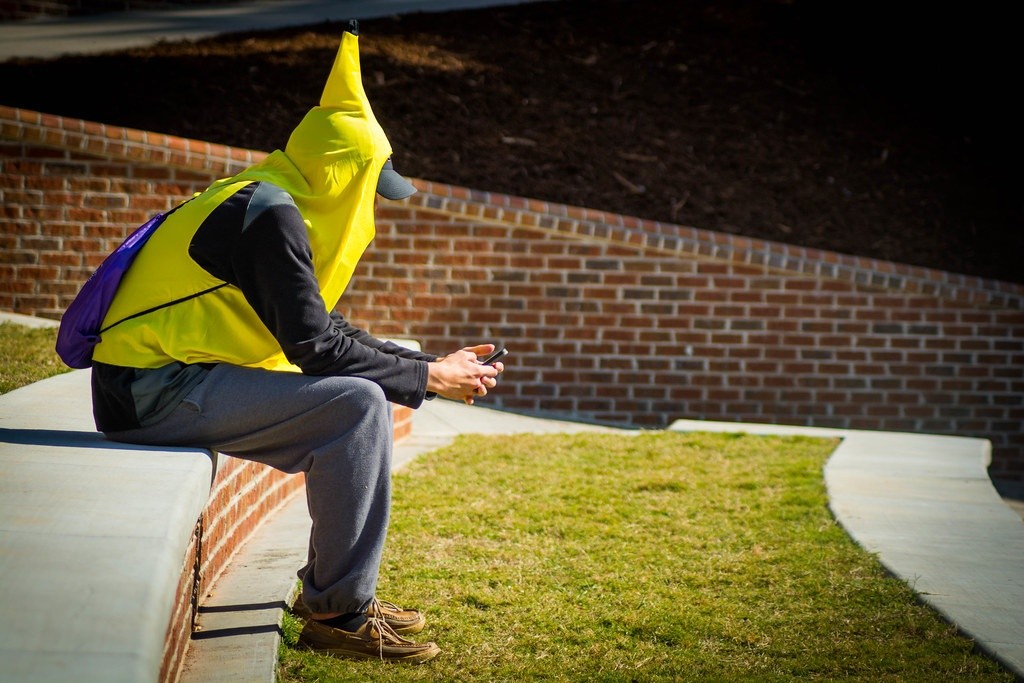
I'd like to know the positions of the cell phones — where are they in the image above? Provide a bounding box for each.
[482,348,509,366]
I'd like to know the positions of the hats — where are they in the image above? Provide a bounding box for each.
[376,157,418,202]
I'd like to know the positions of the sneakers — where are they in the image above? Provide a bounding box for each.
[292,590,425,634]
[295,614,444,666]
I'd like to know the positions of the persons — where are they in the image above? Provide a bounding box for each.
[90,18,511,664]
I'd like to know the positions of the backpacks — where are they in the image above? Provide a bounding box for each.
[52,190,233,369]
